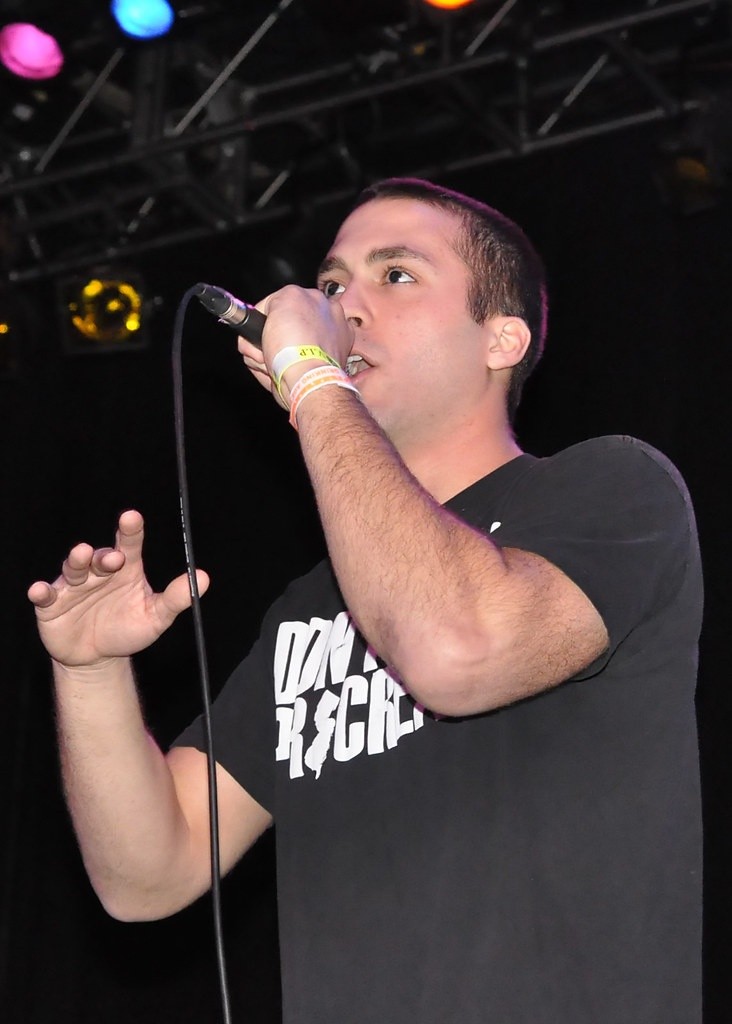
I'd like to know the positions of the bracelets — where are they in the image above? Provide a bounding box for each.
[269,344,363,434]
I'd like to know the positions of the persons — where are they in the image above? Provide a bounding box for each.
[26,175,704,1024]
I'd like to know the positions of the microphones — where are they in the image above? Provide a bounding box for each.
[195,282,267,349]
